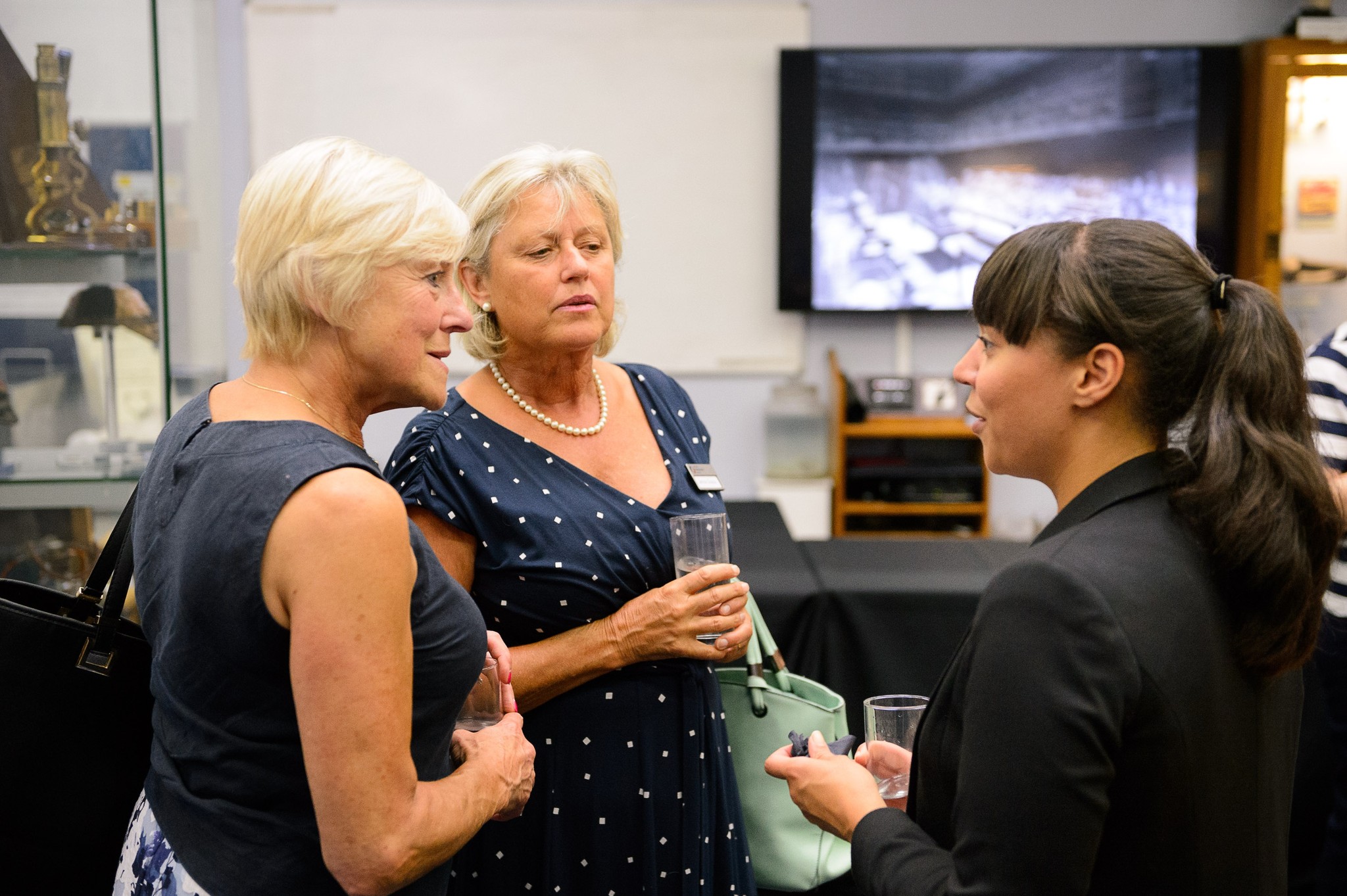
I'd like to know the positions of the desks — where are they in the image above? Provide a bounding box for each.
[717,502,1034,733]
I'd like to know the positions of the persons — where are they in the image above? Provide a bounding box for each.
[763,218,1346,896]
[110,135,536,896]
[384,147,753,895]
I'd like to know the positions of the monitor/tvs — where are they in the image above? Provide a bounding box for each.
[777,43,1241,312]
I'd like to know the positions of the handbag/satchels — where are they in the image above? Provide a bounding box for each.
[0,482,140,896]
[704,574,854,891]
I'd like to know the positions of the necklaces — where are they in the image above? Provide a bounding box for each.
[490,361,608,436]
[242,374,364,448]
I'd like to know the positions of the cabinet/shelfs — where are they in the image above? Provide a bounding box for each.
[0,0,170,611]
[828,357,996,546]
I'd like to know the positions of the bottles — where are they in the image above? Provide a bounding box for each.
[23,144,100,241]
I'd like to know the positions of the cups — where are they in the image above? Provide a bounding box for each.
[862,694,930,814]
[450,659,505,766]
[668,514,735,639]
